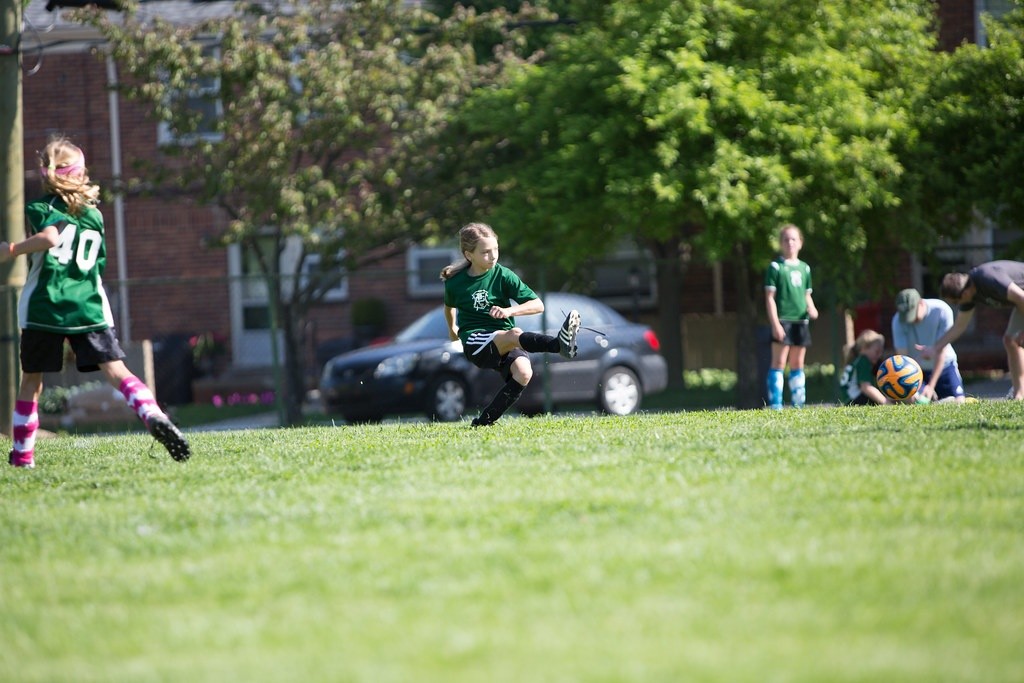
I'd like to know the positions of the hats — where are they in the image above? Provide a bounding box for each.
[894,288,920,324]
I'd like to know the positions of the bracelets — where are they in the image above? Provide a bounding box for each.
[10,242,16,258]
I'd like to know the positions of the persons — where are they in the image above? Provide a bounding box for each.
[840,330,893,405]
[892,289,965,401]
[0,142,191,467]
[764,225,818,409]
[440,223,581,426]
[915,260,1024,401]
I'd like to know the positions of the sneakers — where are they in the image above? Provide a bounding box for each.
[558,309,582,358]
[471,418,478,428]
[9,451,35,469]
[145,415,191,463]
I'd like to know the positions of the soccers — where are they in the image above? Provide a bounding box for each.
[876,355,923,401]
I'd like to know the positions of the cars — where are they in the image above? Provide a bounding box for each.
[319,289,668,426]
